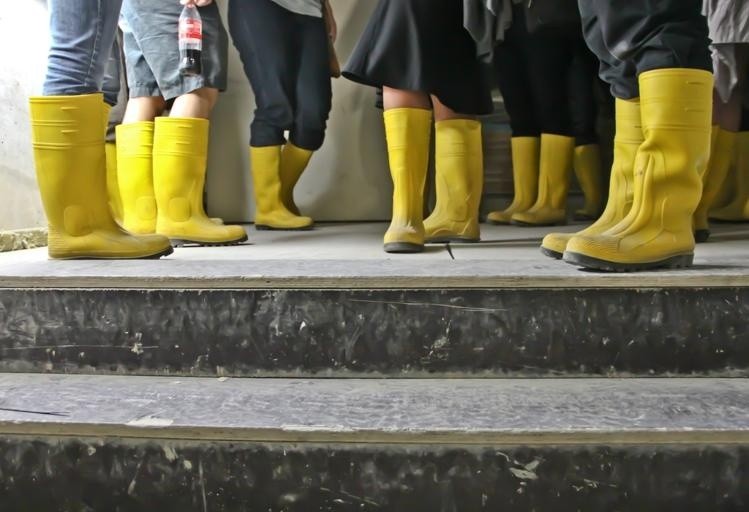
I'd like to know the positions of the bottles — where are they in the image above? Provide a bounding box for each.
[177,1,204,77]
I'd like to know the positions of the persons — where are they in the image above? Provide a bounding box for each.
[342,1,749,272]
[29,0,341,260]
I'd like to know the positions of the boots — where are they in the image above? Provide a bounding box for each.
[693,124,737,242]
[512,132,576,227]
[539,96,645,260]
[28,93,249,260]
[572,143,608,221]
[383,107,433,253]
[486,136,540,225]
[563,68,714,273]
[423,119,484,243]
[252,141,314,229]
[708,131,749,222]
